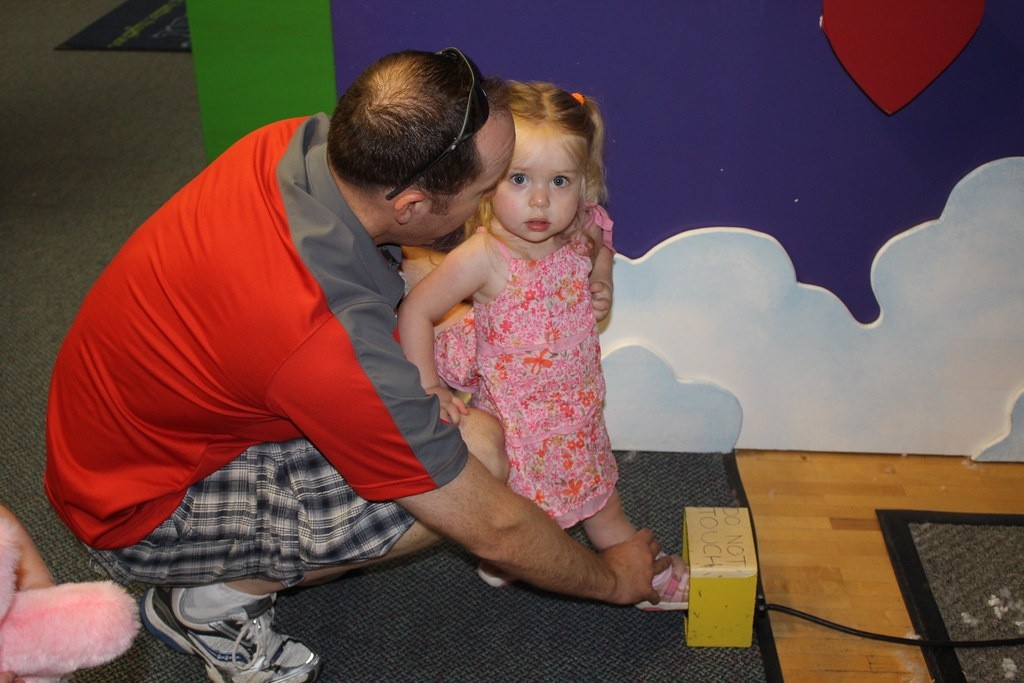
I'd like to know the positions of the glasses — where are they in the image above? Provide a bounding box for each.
[385,48,489,201]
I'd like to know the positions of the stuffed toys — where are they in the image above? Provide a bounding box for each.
[0,517,142,683]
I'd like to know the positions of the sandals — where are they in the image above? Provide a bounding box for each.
[635,555,690,611]
[478,555,521,590]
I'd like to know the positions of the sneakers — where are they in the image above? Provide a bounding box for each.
[140,583,320,683]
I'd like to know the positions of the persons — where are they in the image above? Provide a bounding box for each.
[44,46,691,683]
[0,502,57,683]
